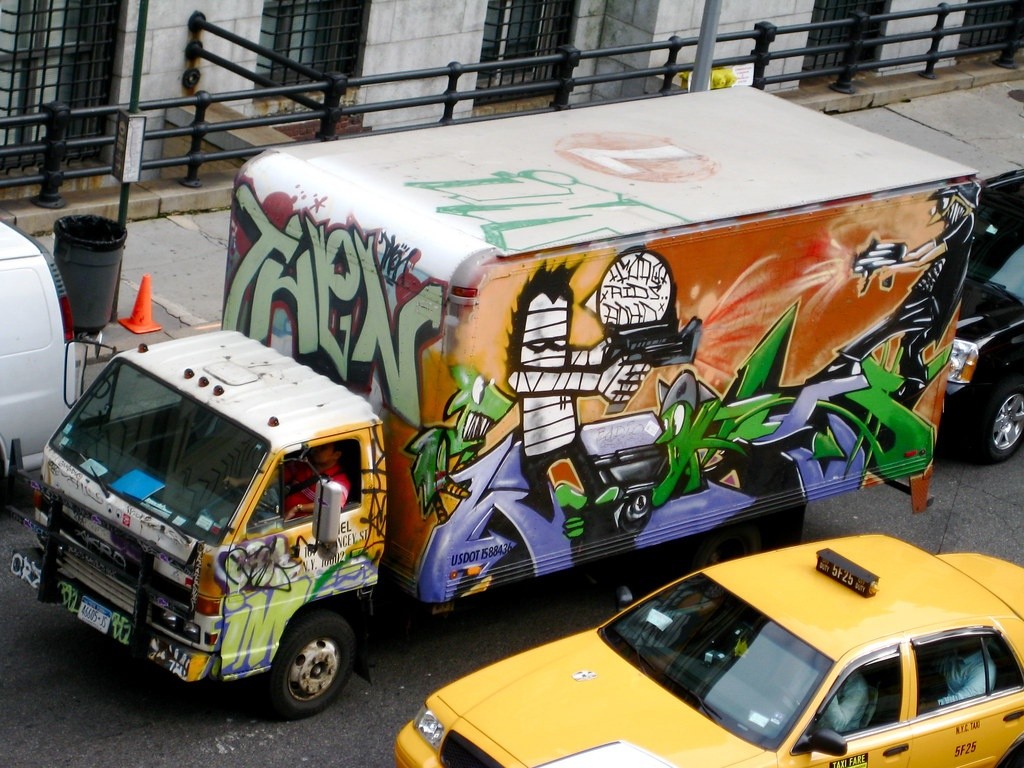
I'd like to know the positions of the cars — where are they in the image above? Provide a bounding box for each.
[396,529,1024,768]
[940,167,1024,463]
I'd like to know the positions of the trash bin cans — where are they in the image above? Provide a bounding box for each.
[52,214,128,332]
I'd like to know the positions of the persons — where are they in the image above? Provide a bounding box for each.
[816,669,870,734]
[915,642,997,714]
[222,443,351,521]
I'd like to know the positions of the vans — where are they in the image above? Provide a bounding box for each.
[0,218,76,498]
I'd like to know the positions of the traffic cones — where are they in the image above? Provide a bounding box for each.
[117,273,161,336]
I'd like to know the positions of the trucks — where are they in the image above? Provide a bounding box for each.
[4,84,982,720]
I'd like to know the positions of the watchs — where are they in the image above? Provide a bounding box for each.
[296,503,303,512]
